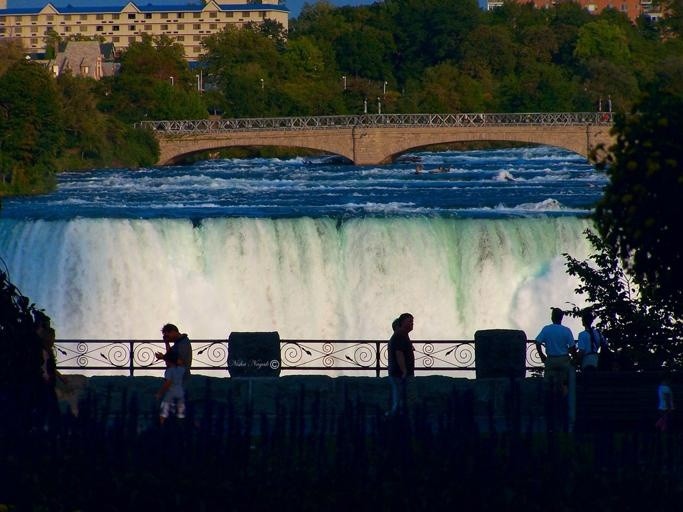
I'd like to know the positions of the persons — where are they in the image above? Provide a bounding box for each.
[387,313,416,417]
[535,308,576,394]
[657,372,675,438]
[154,348,186,426]
[154,324,192,414]
[576,312,607,370]
